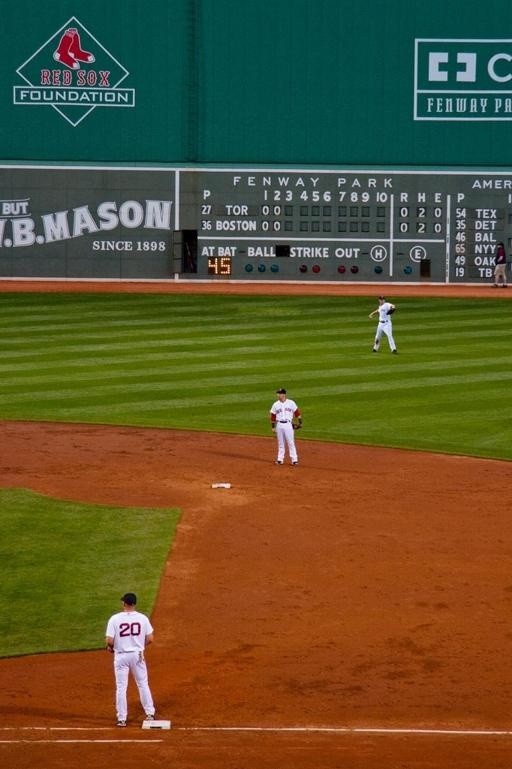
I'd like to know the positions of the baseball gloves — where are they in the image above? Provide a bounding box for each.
[386,308,395,315]
[292,424,302,429]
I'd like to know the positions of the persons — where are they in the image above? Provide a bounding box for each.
[270,388,301,465]
[369,296,397,352]
[105,593,155,727]
[490,241,508,288]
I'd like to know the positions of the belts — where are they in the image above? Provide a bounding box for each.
[277,420,290,424]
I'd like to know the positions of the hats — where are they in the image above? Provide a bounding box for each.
[378,295,385,301]
[276,388,287,394]
[120,593,136,605]
